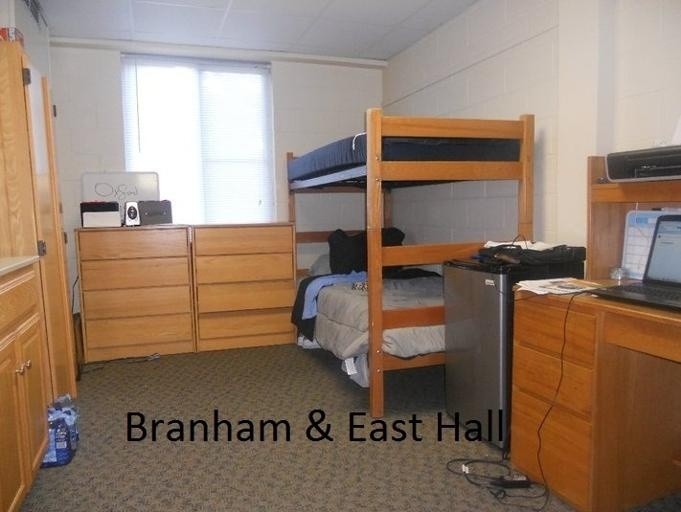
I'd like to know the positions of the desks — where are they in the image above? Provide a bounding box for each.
[506,145,681,510]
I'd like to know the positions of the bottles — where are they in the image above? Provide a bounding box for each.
[41,405,78,467]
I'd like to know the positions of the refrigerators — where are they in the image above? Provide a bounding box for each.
[441,254,588,452]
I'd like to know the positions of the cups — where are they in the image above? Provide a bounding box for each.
[607,266,628,288]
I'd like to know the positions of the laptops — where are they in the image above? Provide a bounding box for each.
[586,214,681,310]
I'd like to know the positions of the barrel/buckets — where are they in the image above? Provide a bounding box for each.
[56,405,79,452]
[39,407,73,470]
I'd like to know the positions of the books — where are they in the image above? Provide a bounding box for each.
[534,277,605,296]
[515,278,560,295]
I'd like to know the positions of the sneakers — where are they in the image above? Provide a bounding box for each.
[297,332,321,349]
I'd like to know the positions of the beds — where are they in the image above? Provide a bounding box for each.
[284,106,534,417]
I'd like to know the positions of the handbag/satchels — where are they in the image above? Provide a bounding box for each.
[479,245,586,265]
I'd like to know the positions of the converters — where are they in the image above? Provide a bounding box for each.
[499,475,528,487]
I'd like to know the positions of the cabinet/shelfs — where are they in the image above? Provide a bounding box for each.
[73,224,300,364]
[0,40,78,512]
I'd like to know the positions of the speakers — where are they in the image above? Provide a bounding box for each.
[124,201,141,226]
